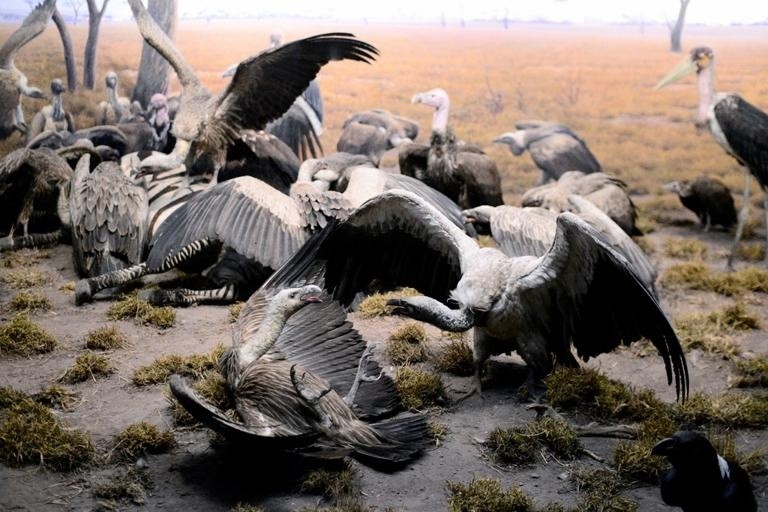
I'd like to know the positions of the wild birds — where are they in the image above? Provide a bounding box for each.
[653,45,768,274]
[2,31,641,306]
[173,188,688,477]
[650,429,758,512]
[660,176,738,233]
